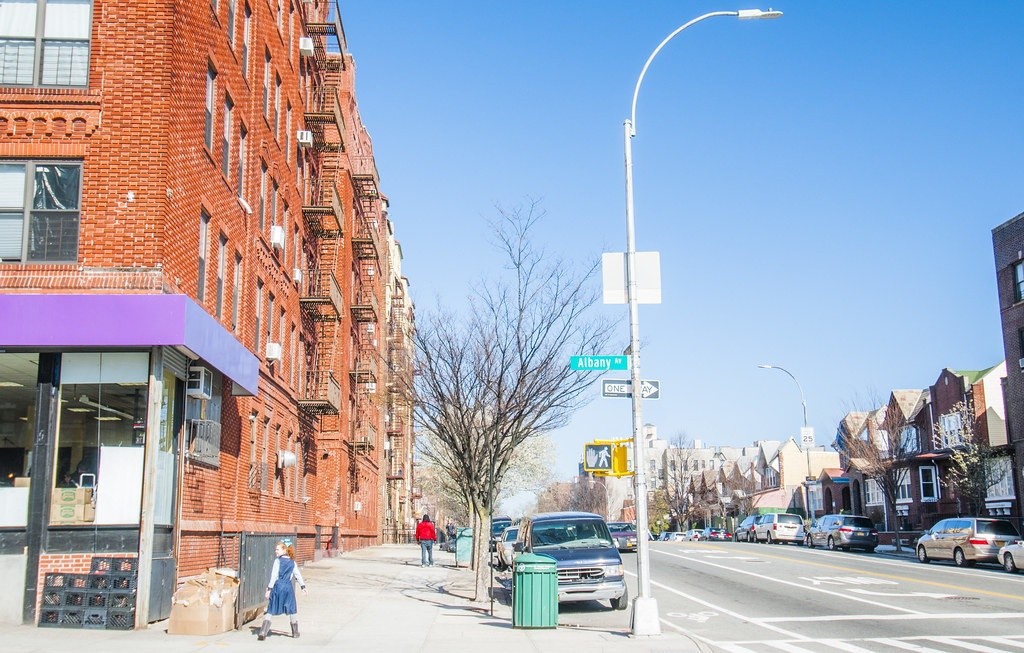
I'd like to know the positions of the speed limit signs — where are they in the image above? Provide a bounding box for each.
[801,427,815,446]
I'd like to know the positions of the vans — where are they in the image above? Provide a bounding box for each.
[753,513,805,546]
[915,517,1022,567]
[514,511,628,610]
[807,514,879,553]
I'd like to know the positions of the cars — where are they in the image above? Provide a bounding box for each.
[734,515,762,543]
[606,522,638,552]
[489,515,513,552]
[650,532,686,542]
[998,541,1024,574]
[699,527,732,541]
[495,526,519,571]
[683,529,705,541]
[511,527,577,563]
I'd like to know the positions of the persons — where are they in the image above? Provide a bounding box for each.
[416,514,437,568]
[257,538,307,640]
[445,519,455,539]
[58,458,92,487]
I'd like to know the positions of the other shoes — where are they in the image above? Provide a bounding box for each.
[429,563,435,567]
[422,564,427,567]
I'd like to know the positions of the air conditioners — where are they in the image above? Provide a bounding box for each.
[354,502,362,511]
[297,131,313,148]
[187,366,213,400]
[366,382,376,394]
[299,38,314,56]
[384,441,390,450]
[269,225,285,249]
[293,269,303,284]
[265,342,283,365]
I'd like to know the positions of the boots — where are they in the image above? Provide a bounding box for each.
[291,622,300,637]
[259,620,272,639]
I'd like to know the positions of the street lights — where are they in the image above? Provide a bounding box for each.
[623,9,785,637]
[589,481,610,522]
[758,365,815,524]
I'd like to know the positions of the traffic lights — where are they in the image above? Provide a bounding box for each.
[583,443,613,472]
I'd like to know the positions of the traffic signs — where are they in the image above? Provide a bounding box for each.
[602,379,660,400]
[570,356,631,371]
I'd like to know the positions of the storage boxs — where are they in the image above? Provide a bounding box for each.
[49,487,93,526]
[38,557,139,630]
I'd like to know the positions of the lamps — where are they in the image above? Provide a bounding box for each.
[367,323,375,333]
[79,395,134,420]
